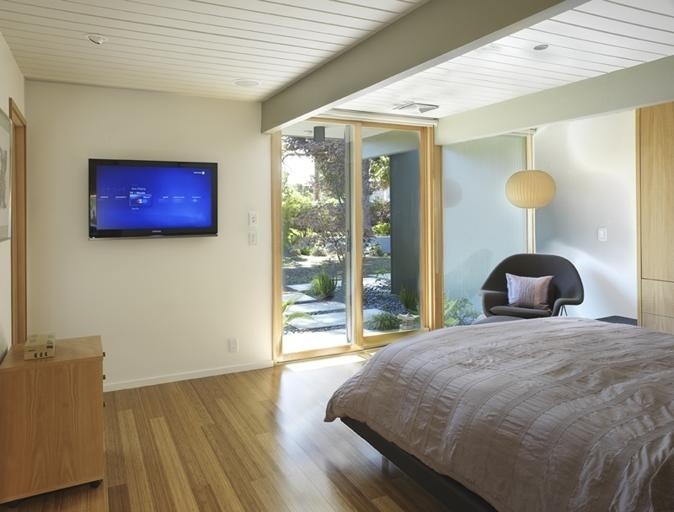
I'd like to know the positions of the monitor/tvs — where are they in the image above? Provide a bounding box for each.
[88,158,219,239]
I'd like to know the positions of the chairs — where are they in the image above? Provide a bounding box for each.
[481,253,585,321]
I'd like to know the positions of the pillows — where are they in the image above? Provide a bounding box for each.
[504,272,554,310]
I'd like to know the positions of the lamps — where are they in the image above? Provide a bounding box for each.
[504,128,557,209]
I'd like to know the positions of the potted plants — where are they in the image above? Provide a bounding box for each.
[371,223,392,253]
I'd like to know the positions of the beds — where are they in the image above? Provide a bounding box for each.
[341,318,674,511]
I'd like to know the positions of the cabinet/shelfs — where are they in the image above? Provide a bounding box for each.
[3,334,107,501]
